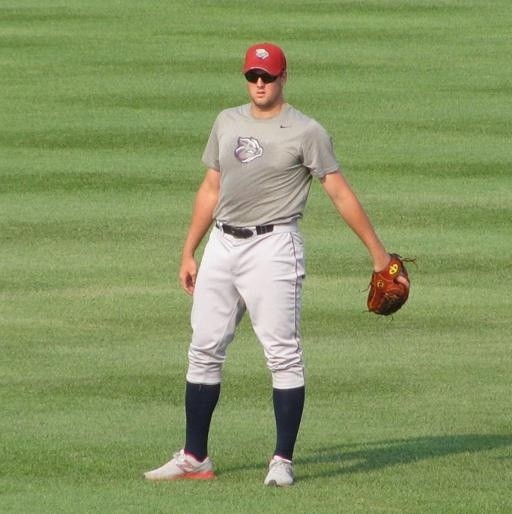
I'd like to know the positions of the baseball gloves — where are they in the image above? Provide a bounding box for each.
[368,252,411,315]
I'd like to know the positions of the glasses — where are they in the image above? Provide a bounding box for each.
[245,71,277,84]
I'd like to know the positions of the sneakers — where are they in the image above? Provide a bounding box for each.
[264,455,297,487]
[142,448,215,483]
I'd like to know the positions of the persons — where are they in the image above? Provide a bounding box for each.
[143,43,410,487]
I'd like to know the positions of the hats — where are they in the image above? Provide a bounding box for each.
[241,42,287,77]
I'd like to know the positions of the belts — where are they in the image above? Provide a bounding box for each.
[215,222,274,238]
